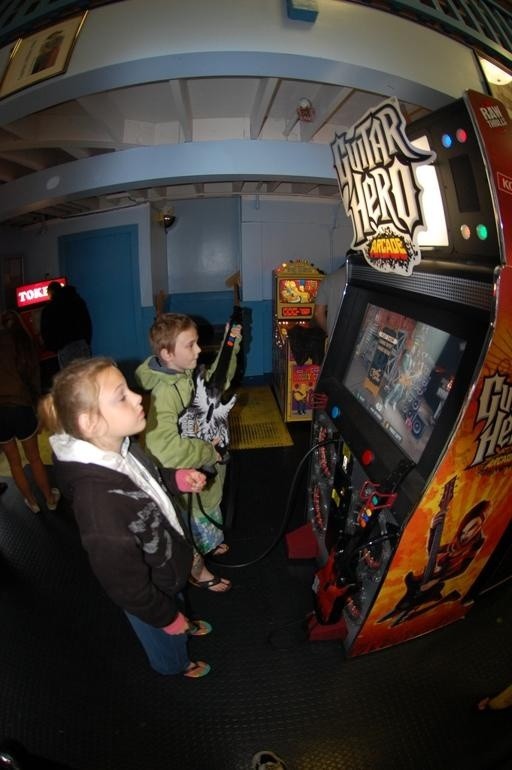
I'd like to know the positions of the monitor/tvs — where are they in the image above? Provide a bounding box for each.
[340,301,469,466]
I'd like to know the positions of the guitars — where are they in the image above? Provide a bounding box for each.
[178,306,243,464]
[312,459,415,625]
[396,475,457,610]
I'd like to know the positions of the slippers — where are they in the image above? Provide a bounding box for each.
[189,575,231,593]
[215,543,229,554]
[189,620,212,636]
[184,660,211,678]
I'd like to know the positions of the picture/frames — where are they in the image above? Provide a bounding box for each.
[2,10,89,98]
[1,257,25,294]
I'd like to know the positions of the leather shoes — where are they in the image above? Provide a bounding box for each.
[0,481,7,493]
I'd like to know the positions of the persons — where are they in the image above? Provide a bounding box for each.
[40,281,93,370]
[312,263,350,351]
[383,344,420,411]
[37,355,213,679]
[132,314,244,594]
[0,295,61,514]
[472,684,512,715]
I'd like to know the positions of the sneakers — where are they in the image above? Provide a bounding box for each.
[46,488,61,511]
[250,750,288,770]
[23,498,39,514]
